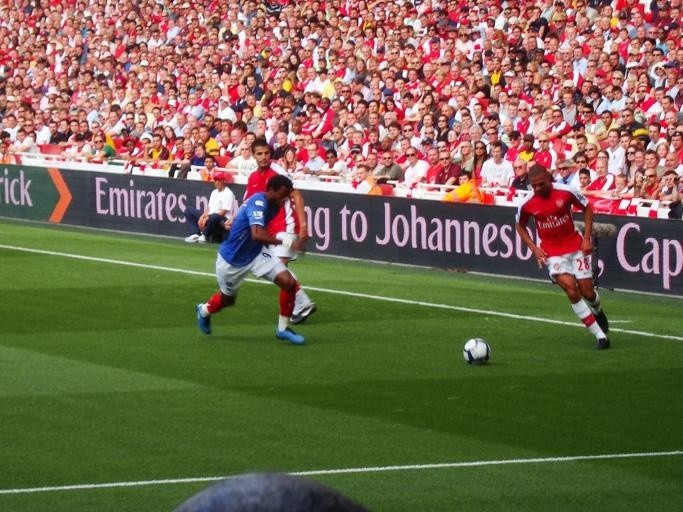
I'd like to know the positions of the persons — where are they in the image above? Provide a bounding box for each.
[221,135,318,326]
[510,164,612,348]
[193,174,308,345]
[0,0,683,221]
[182,171,241,245]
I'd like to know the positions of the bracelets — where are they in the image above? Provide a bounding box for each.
[280,237,293,249]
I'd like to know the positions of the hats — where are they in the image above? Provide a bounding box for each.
[214,173,225,181]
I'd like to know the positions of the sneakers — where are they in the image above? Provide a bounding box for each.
[594,337,611,351]
[591,309,609,331]
[195,303,213,335]
[275,327,305,345]
[184,233,208,243]
[290,303,316,324]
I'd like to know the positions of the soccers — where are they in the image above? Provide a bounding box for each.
[463,337,491,364]
